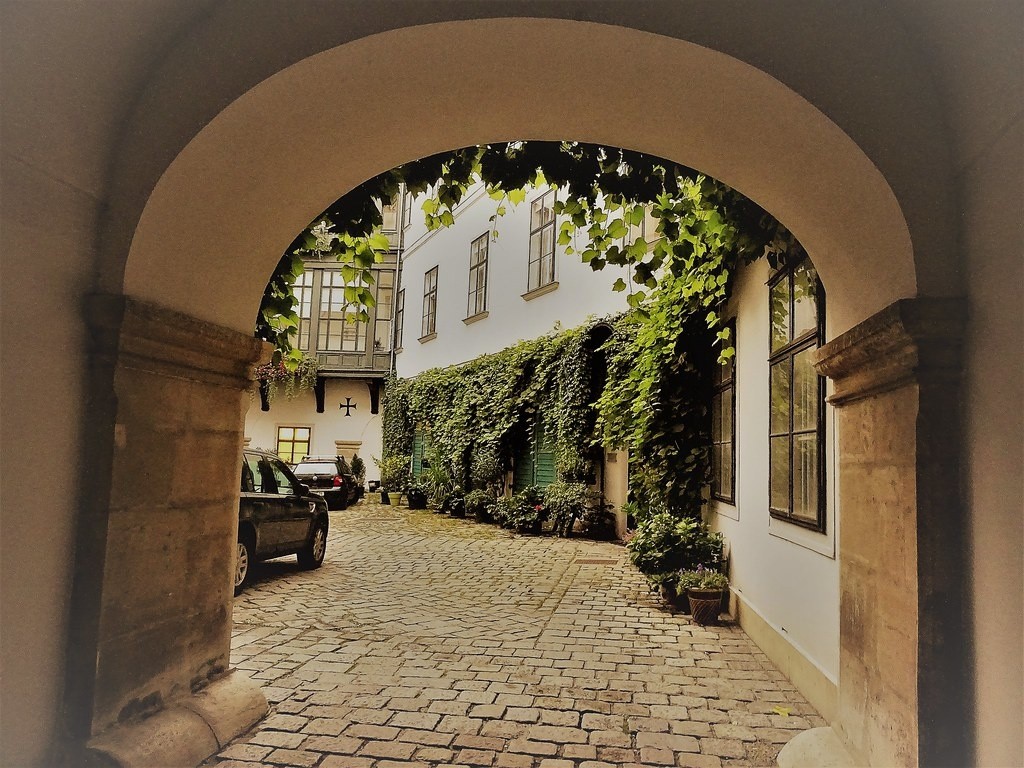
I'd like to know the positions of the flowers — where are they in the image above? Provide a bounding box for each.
[253,358,303,382]
[677,561,730,596]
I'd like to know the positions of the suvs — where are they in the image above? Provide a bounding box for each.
[234,449,330,595]
[290,455,361,510]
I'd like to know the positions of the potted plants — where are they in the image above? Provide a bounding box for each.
[370,453,549,536]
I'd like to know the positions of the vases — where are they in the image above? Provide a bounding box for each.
[686,587,723,624]
